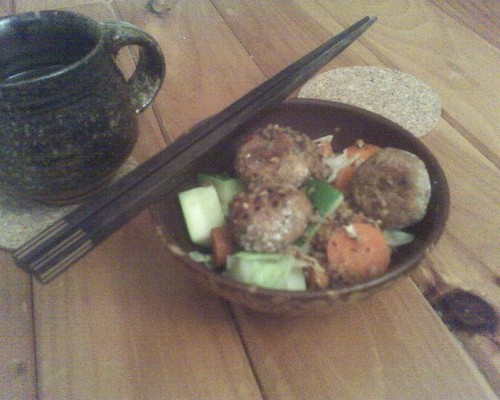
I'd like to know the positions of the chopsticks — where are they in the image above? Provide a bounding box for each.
[12,14,378,283]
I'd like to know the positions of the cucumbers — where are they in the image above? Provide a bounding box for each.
[179,171,348,292]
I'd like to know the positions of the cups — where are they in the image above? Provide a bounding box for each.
[0,9,168,207]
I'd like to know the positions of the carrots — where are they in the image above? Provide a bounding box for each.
[212,138,390,289]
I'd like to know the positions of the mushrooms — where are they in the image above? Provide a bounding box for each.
[230,124,433,254]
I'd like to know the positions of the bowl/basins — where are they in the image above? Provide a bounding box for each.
[149,94,455,317]
